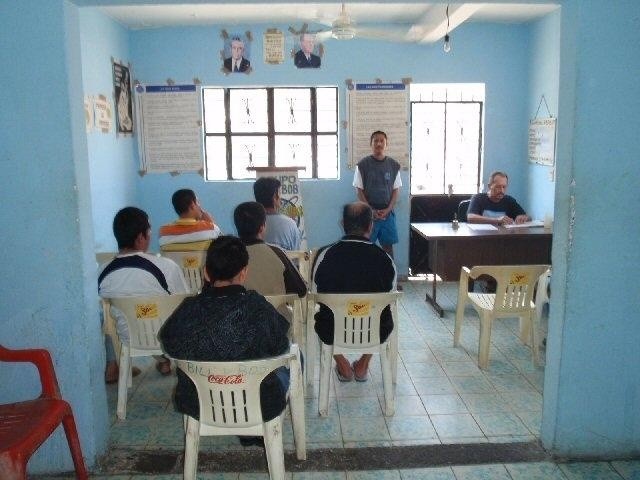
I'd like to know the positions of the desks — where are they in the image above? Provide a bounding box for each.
[411,221,552,317]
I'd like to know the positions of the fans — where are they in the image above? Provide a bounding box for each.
[283,2,411,41]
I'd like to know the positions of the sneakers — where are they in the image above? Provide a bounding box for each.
[155,359,171,373]
[105,365,141,383]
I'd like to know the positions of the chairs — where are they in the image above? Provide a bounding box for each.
[457,200,471,222]
[453,263,549,372]
[263,294,304,347]
[0,346,87,480]
[94,250,311,367]
[308,292,401,418]
[165,345,306,480]
[101,293,187,420]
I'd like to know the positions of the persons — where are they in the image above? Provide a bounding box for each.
[156,234,305,447]
[293,33,322,69]
[232,201,308,323]
[466,171,532,226]
[98,206,188,383]
[156,188,223,245]
[253,177,303,272]
[310,201,398,382]
[352,131,403,291]
[223,37,250,73]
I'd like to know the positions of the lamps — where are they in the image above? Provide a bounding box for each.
[443,3,450,52]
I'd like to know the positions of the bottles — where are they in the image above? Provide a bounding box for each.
[447,184,453,198]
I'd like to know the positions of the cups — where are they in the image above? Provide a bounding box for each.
[544,215,552,229]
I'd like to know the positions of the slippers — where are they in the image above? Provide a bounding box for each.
[334,364,355,382]
[351,360,369,382]
